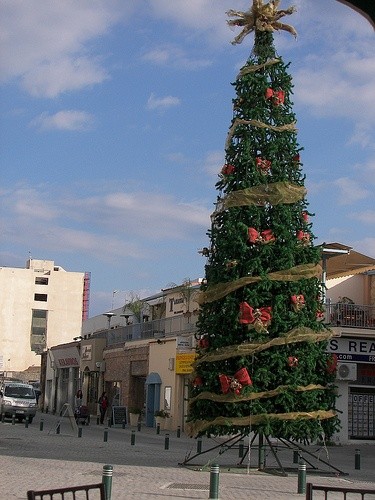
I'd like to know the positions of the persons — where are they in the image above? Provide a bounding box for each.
[36,393,39,404]
[98,392,109,424]
[74,390,84,419]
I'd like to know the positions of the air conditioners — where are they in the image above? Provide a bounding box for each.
[334,362,358,382]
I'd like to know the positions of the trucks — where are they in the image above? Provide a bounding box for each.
[0,382,39,423]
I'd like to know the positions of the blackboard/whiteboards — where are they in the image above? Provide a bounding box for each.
[111,406,127,425]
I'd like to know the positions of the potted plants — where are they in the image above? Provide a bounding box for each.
[153,409,170,432]
[129,408,141,426]
